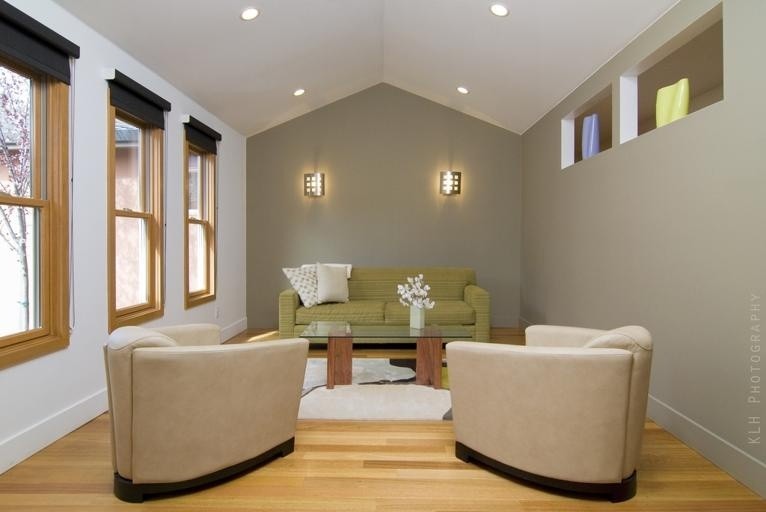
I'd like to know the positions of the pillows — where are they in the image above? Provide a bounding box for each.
[316,261,350,304]
[281,266,318,309]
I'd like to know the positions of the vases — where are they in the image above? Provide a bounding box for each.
[656,78,689,127]
[582,113,600,159]
[408,305,425,329]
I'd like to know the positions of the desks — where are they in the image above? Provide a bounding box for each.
[300,320,473,390]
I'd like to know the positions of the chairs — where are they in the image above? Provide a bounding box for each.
[444,323,655,503]
[101,321,310,500]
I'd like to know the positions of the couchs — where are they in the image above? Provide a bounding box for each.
[277,265,491,342]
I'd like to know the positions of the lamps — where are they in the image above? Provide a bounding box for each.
[302,172,326,198]
[439,170,462,196]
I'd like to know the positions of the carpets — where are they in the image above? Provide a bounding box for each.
[297,354,453,424]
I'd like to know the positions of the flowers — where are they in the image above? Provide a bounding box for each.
[396,273,435,309]
